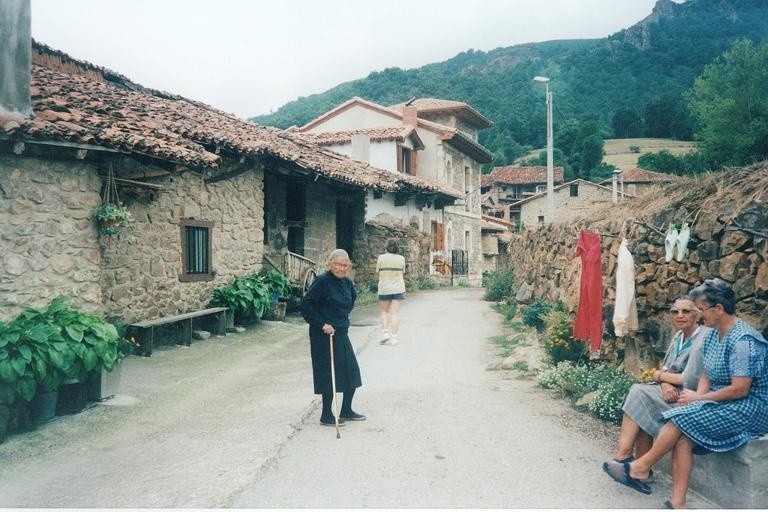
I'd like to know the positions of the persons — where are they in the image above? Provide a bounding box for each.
[374,238,408,348]
[301,248,367,427]
[613,296,713,484]
[600,275,768,509]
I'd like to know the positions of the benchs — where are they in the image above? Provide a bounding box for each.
[130,306,230,357]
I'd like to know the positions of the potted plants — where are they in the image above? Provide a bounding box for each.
[207,270,293,331]
[95,201,134,231]
[0,293,133,443]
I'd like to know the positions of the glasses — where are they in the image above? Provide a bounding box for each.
[697,305,716,312]
[669,307,697,315]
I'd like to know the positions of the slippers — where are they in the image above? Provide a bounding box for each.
[603,460,652,495]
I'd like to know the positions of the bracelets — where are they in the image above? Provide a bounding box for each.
[658,369,666,382]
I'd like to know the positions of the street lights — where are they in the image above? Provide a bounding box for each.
[533,76,554,224]
[613,170,622,203]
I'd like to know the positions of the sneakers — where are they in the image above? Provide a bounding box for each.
[613,456,654,483]
[319,416,345,426]
[379,334,399,345]
[340,412,365,420]
